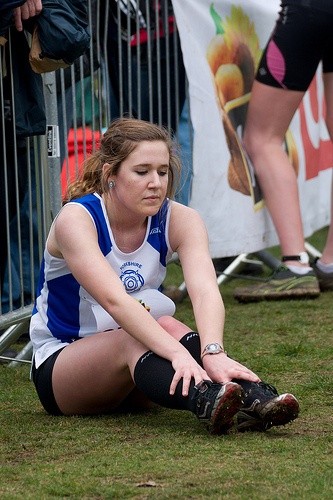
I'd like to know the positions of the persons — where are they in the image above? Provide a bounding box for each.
[0,0,188,309]
[30,115,300,431]
[226,0,333,304]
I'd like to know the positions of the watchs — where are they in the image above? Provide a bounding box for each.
[201,343,227,357]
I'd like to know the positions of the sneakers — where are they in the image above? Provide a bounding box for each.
[235,381,300,432]
[191,380,244,436]
[233,256,333,304]
